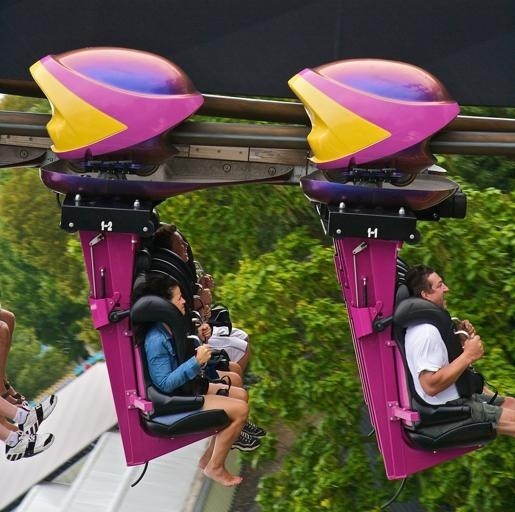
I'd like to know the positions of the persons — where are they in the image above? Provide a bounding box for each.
[482,386,500,396]
[404,267,514,438]
[140,224,267,489]
[0,307,58,463]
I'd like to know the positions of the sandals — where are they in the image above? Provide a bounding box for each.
[2,381,25,404]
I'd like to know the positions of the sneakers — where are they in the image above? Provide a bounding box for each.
[230,430,262,453]
[5,429,55,461]
[17,394,58,436]
[242,423,268,439]
